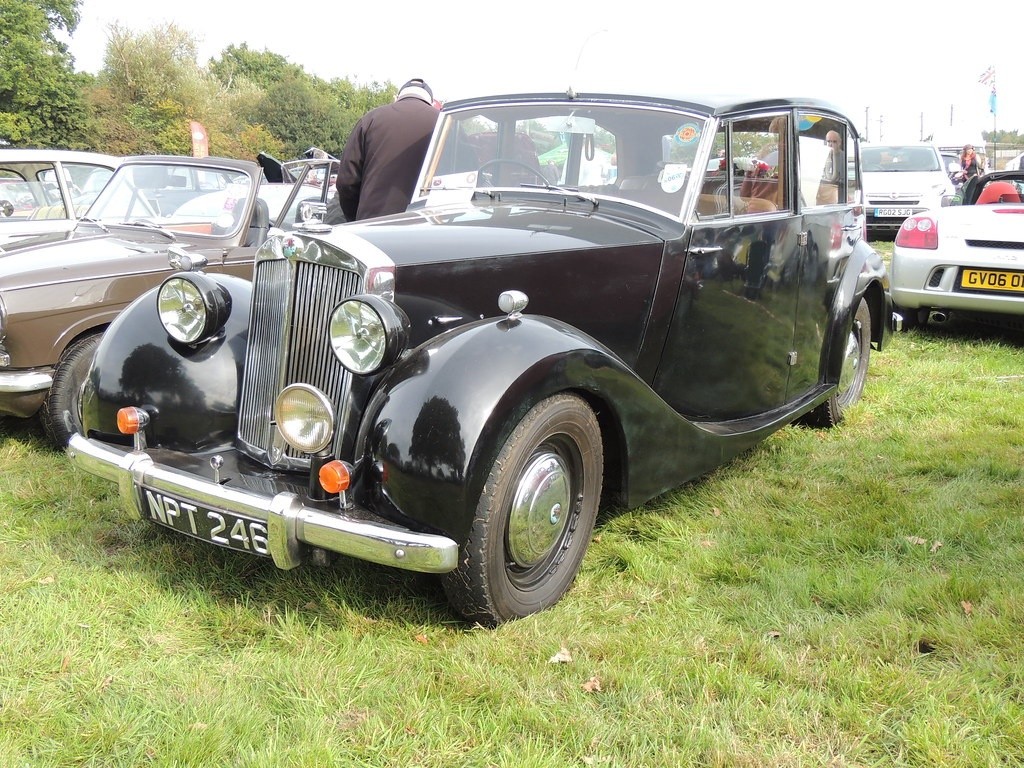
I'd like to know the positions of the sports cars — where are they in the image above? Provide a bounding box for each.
[890,171,1024,332]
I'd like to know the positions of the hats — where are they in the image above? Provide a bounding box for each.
[396,78,434,104]
[963,144,974,151]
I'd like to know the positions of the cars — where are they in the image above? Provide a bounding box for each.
[859,143,958,232]
[82,155,341,228]
[0,155,333,452]
[64,83,903,627]
[1,149,159,245]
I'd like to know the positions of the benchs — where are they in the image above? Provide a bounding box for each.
[620,176,840,211]
[27,206,90,220]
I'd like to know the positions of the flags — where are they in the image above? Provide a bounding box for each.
[978,65,997,114]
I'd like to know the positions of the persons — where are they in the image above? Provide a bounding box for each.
[752,159,769,178]
[960,144,981,183]
[717,224,771,279]
[738,117,787,213]
[335,78,488,222]
[719,150,725,166]
[823,130,845,183]
[543,159,560,186]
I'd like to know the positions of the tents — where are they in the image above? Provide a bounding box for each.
[538,143,569,165]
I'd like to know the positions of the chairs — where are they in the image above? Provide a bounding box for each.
[975,182,1022,205]
[226,197,270,248]
[670,195,775,217]
